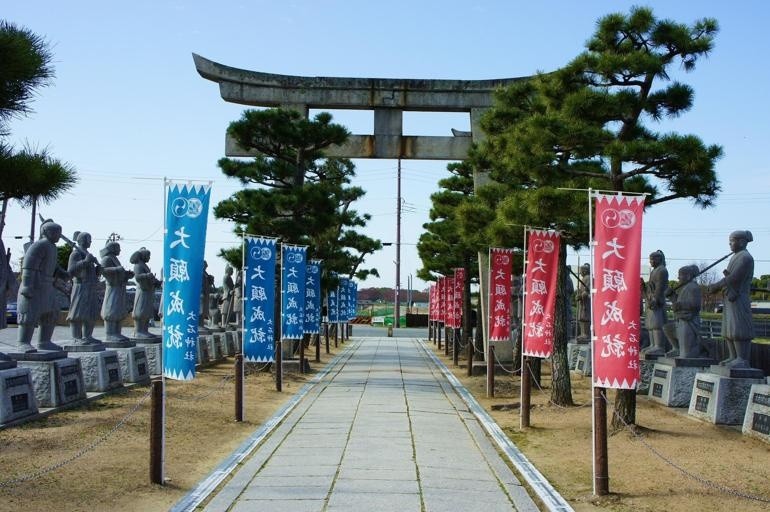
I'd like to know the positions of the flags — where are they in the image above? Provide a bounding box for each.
[304,264,321,333]
[281,243,308,338]
[243,236,277,363]
[428,192,648,390]
[163,185,212,380]
[328,272,358,323]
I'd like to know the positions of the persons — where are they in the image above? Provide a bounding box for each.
[1,215,246,361]
[562,226,758,367]
